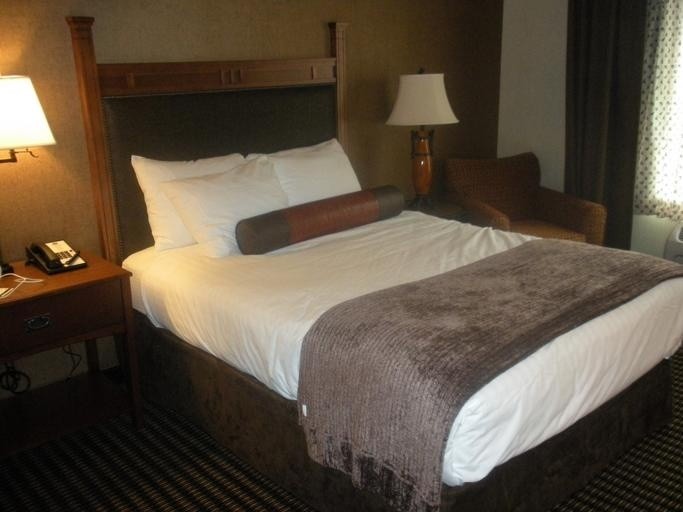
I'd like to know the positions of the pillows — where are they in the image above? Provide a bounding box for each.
[128,135,403,262]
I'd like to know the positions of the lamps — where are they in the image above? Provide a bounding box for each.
[0,74,58,274]
[385,70,459,211]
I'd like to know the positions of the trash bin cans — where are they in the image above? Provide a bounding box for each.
[663,223,683,261]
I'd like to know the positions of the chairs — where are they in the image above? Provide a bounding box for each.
[448,149,608,244]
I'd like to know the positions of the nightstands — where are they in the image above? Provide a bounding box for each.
[403,197,471,225]
[1,249,146,438]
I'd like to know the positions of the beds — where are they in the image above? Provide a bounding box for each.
[63,13,683,512]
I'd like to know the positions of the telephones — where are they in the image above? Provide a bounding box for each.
[25,240,87,275]
